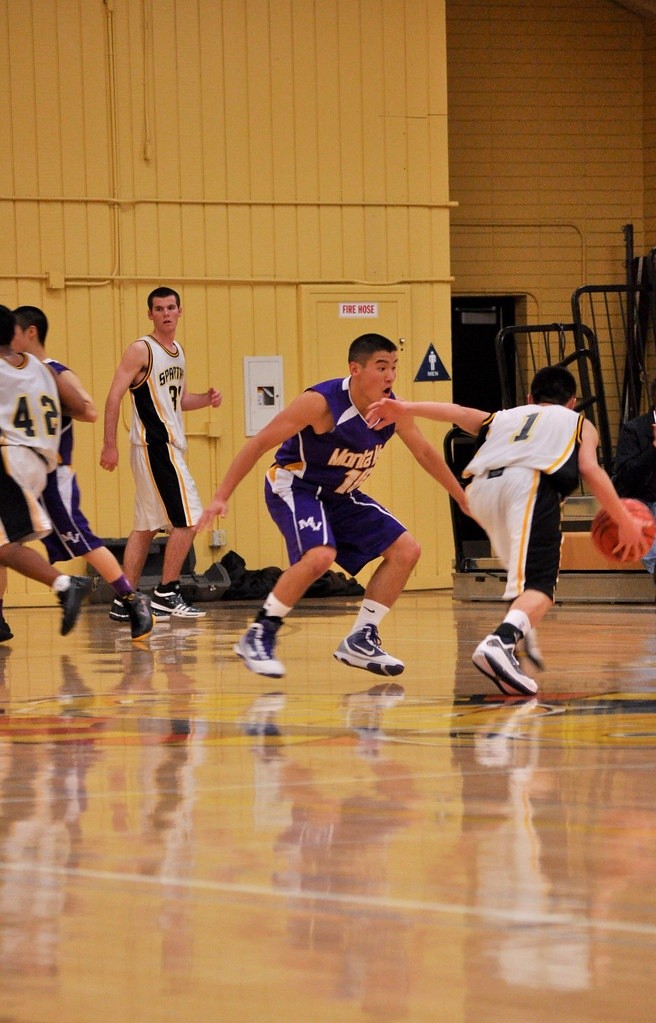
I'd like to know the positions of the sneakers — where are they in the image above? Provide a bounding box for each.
[472,634,538,697]
[231,620,285,677]
[0,617,14,643]
[109,596,130,622]
[519,629,544,673]
[333,624,404,675]
[451,695,538,764]
[56,575,92,636]
[150,584,206,618]
[337,683,403,717]
[124,591,153,639]
[238,692,285,737]
[61,622,204,704]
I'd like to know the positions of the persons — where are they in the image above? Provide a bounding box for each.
[365,366,652,696]
[194,333,474,678]
[99,287,223,621]
[615,379,656,581]
[0,304,156,643]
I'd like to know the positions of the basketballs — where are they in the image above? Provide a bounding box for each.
[590,498,656,562]
